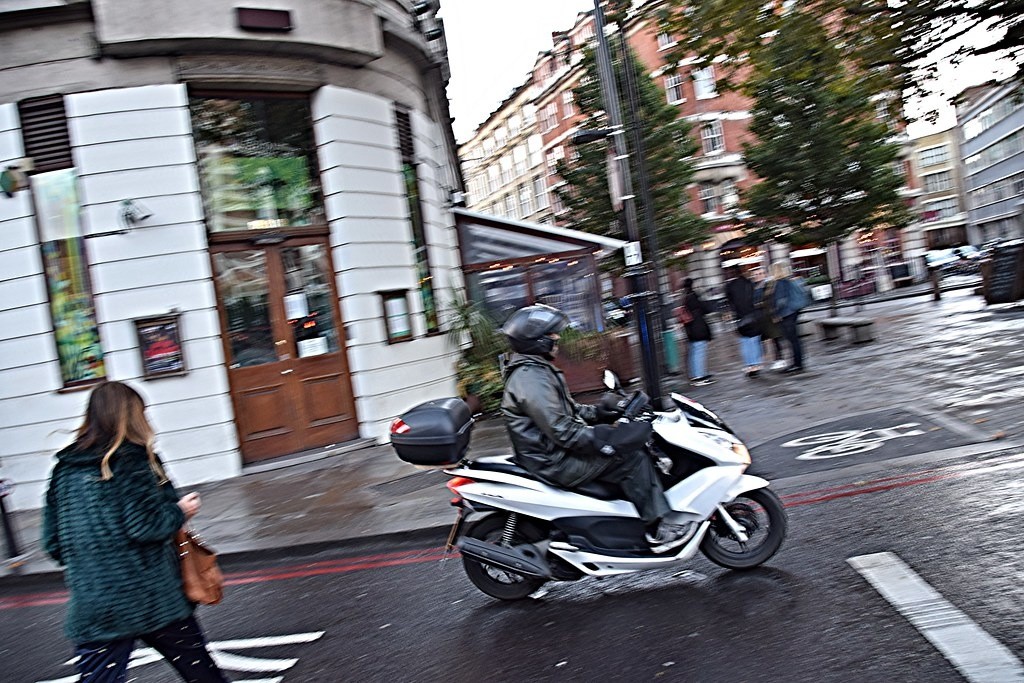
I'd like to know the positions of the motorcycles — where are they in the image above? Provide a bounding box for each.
[390,367,789,603]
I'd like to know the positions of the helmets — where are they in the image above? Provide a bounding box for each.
[500,303,572,356]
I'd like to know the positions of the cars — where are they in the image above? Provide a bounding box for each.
[618,290,656,313]
[981,238,1024,306]
[601,300,629,327]
[923,239,1000,271]
[227,299,320,352]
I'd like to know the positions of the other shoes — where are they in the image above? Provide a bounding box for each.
[786,364,804,374]
[689,378,716,386]
[770,360,788,371]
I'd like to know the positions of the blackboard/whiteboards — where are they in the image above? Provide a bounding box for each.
[985,242,1024,306]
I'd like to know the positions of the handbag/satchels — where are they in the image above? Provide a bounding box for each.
[674,305,693,325]
[784,279,812,311]
[177,523,223,605]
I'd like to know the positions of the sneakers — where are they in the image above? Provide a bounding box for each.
[644,520,698,554]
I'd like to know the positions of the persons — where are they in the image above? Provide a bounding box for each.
[40,380,231,681]
[669,259,808,388]
[498,304,700,550]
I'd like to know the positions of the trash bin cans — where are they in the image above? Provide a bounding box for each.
[661,327,679,375]
